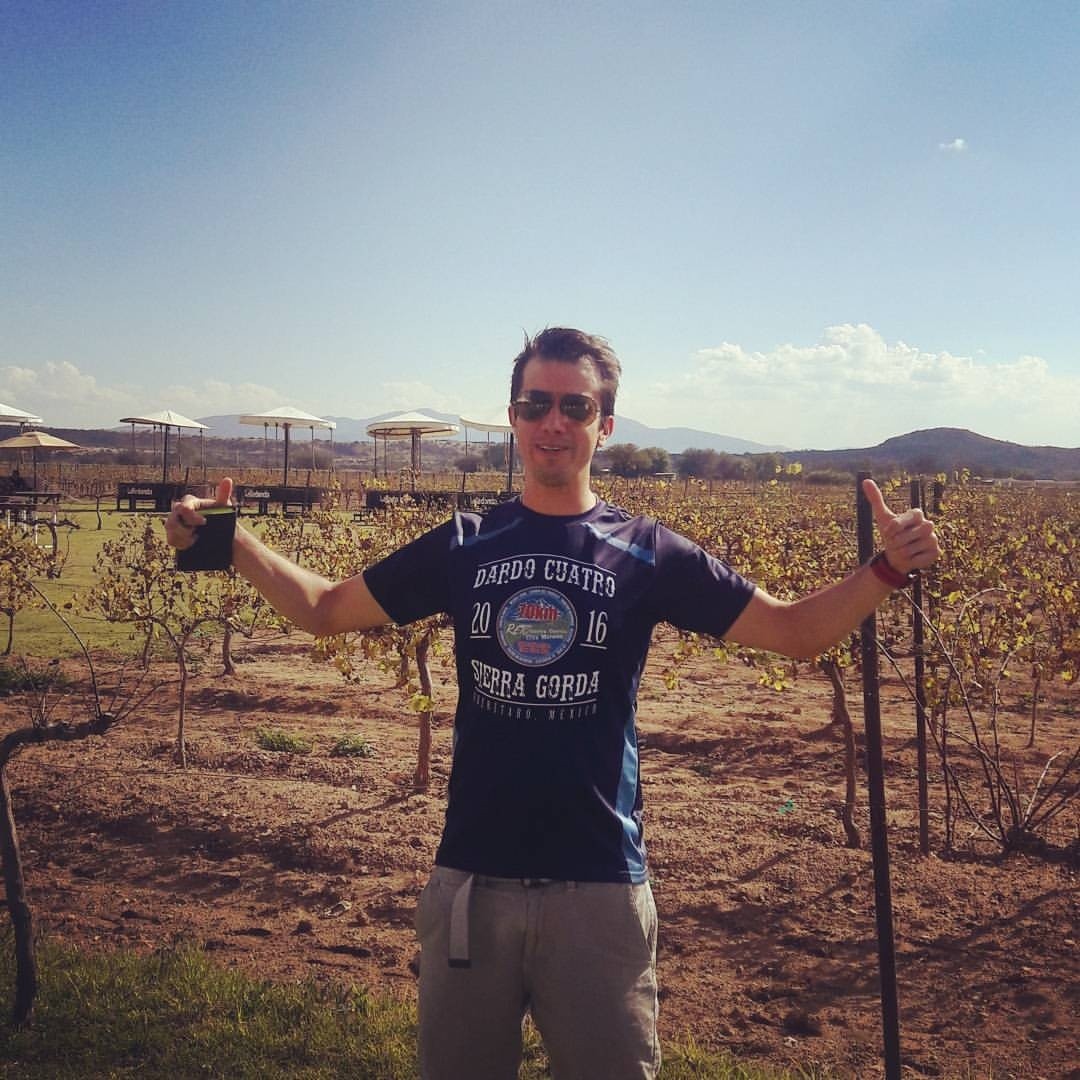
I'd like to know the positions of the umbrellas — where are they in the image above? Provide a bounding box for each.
[0,429,81,490]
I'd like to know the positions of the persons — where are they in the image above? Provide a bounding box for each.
[166,326,943,1080]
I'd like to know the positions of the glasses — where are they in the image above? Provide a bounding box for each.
[511,388,607,426]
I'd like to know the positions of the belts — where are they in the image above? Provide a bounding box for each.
[430,867,559,967]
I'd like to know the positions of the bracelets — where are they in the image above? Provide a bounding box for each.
[870,550,919,590]
